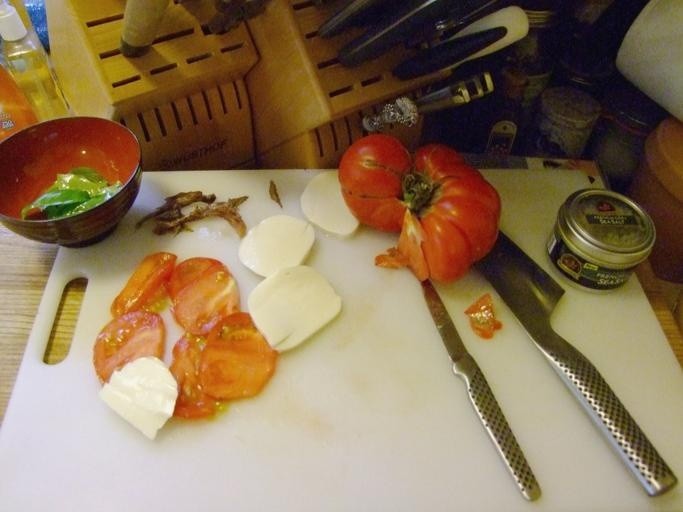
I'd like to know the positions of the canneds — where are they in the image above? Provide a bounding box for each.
[483,0,657,179]
[545,187,656,290]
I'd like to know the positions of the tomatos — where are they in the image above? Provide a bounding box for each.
[93,250,276,419]
[338,131,502,284]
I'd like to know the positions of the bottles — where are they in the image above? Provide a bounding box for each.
[528,86,602,160]
[594,97,656,189]
[0,0,72,143]
[487,6,558,119]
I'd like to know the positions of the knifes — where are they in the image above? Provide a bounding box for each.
[312,0,530,132]
[474,228,678,498]
[421,279,542,503]
[118,0,267,60]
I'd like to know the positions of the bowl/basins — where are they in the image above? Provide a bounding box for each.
[1,115,142,248]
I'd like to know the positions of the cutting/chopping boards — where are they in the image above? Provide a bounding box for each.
[0,169,682,512]
